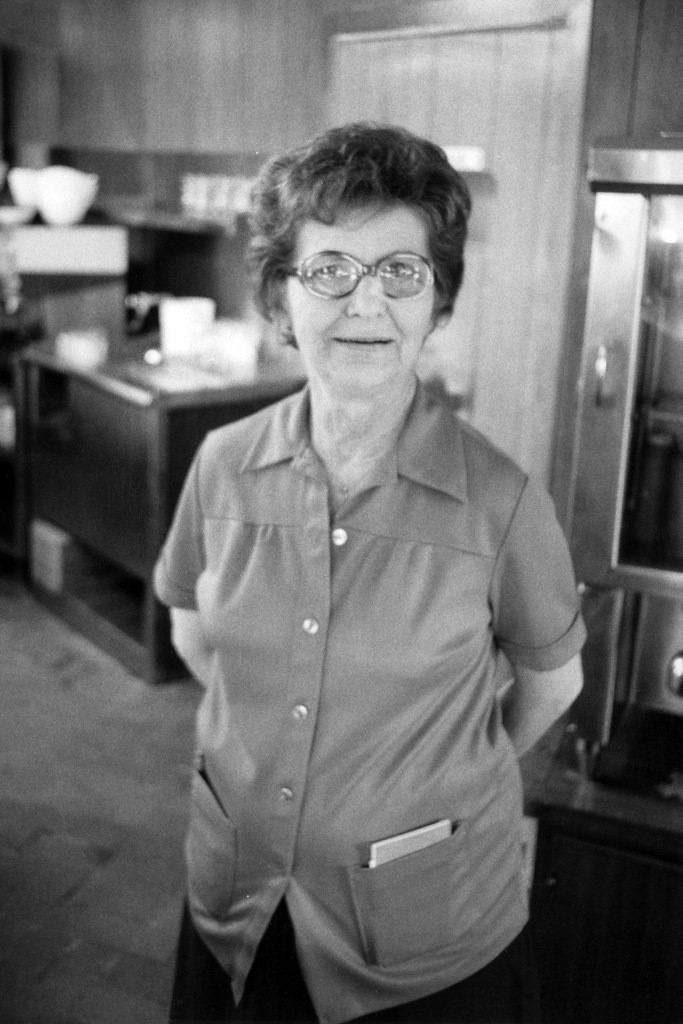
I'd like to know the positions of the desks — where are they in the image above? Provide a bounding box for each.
[9,327,308,690]
[0,221,130,457]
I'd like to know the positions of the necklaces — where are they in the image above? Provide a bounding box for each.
[335,480,354,494]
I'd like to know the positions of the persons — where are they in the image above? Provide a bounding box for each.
[151,128,588,1024]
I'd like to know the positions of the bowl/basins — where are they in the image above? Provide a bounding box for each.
[0,166,100,226]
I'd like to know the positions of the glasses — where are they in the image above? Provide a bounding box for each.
[277,250,433,302]
